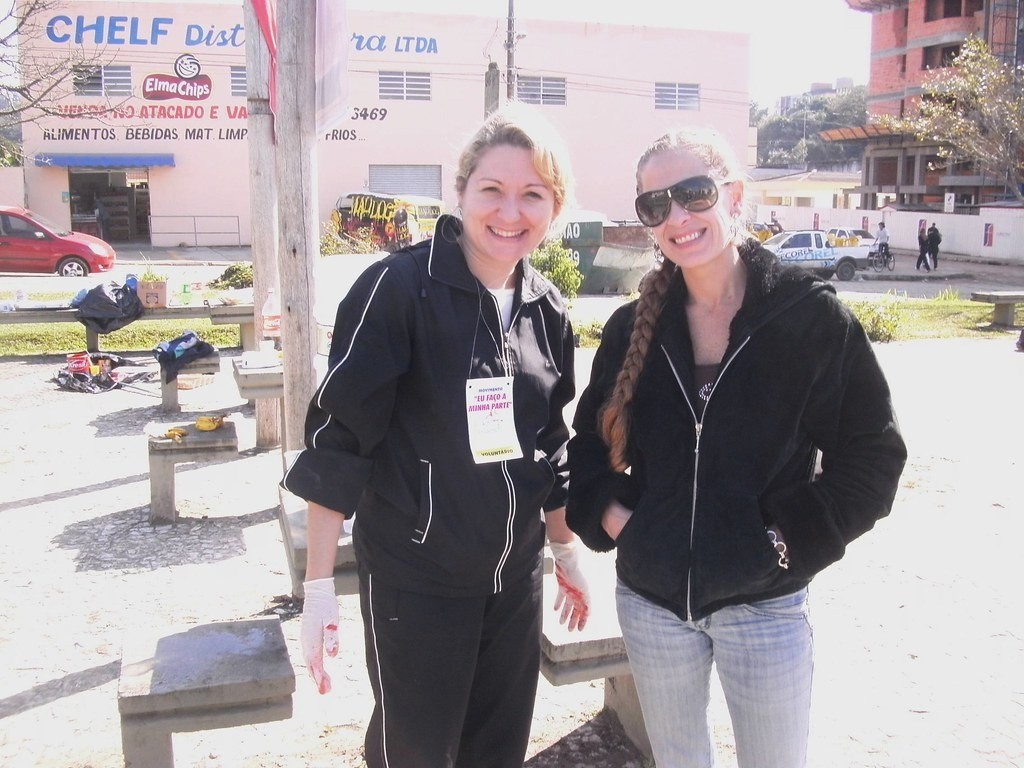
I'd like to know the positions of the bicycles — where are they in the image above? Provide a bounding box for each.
[870,241,895,273]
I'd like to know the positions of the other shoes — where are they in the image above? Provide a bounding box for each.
[926,269,930,273]
[915,265,918,270]
[934,267,937,271]
[890,254,893,261]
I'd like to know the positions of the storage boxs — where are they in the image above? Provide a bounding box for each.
[137,281,166,308]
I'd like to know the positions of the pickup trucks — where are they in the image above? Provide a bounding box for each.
[761,229,870,282]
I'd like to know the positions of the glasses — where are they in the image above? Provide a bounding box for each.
[635,175,732,227]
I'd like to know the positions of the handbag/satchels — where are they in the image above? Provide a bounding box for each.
[152,331,215,384]
[54,351,146,393]
[75,281,145,334]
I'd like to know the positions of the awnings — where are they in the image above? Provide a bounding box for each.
[34,152,175,167]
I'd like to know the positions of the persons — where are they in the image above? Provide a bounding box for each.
[90,197,113,241]
[563,126,909,768]
[872,222,890,268]
[277,96,597,768]
[915,222,942,273]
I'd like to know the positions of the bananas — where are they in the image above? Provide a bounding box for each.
[165,413,226,439]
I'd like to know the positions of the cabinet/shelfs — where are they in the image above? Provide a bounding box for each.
[97,196,131,241]
[136,195,149,226]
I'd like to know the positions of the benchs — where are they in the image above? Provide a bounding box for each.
[0,304,209,352]
[970,291,1024,327]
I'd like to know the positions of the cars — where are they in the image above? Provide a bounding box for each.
[825,227,881,268]
[0,206,115,277]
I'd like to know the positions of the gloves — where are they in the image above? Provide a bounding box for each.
[300,578,339,694]
[550,540,589,631]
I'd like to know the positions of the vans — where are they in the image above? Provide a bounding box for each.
[333,192,444,255]
